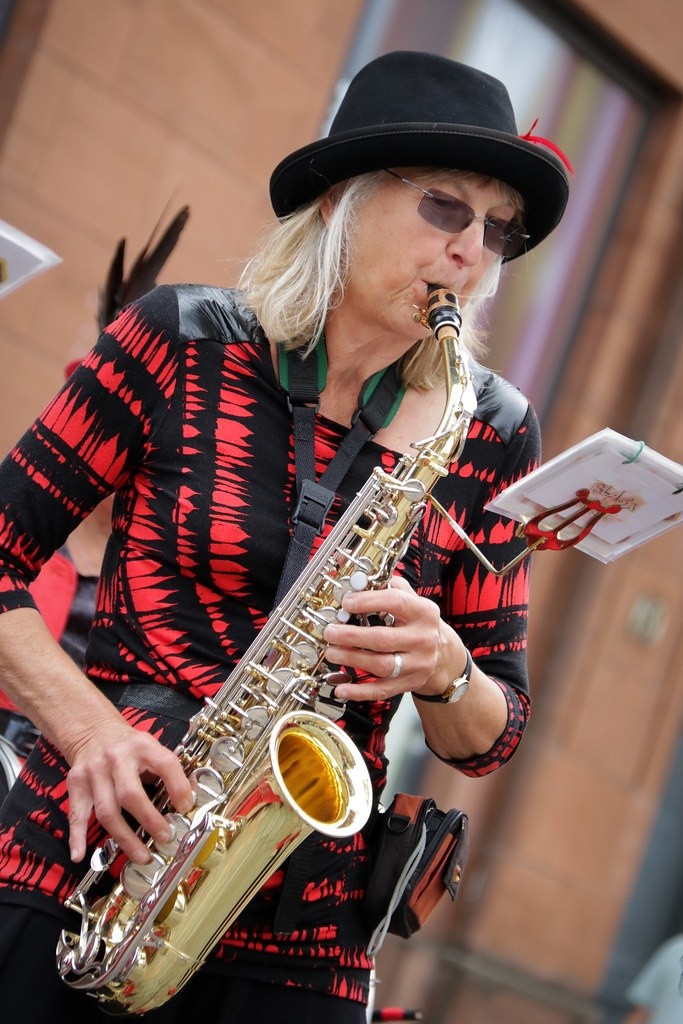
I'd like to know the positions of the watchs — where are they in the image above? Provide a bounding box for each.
[412,647,474,705]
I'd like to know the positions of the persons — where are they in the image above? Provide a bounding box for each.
[0,54,572,1024]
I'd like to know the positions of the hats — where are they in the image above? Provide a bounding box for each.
[269,50,573,266]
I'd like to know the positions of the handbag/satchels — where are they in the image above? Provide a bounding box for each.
[370,788,471,939]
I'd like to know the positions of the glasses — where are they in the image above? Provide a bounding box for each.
[384,169,530,256]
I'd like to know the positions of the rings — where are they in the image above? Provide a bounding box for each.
[390,652,402,679]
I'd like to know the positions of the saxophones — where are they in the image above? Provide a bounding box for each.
[56,283,478,1015]
